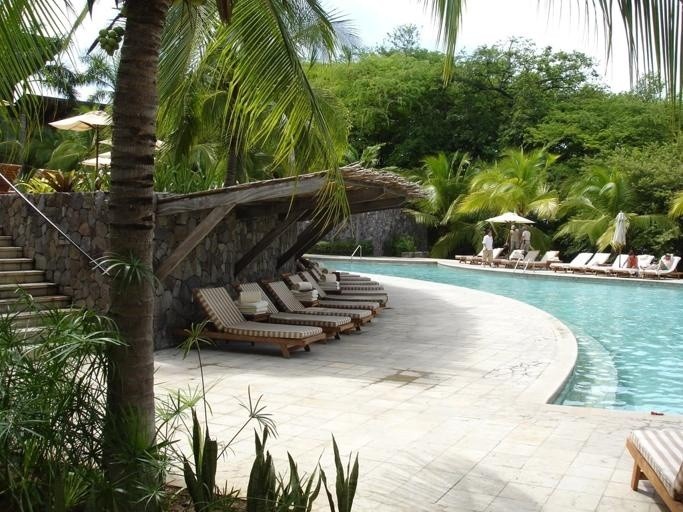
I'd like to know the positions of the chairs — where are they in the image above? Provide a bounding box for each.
[184,255,388,357]
[626,427,683,512]
[455,247,683,282]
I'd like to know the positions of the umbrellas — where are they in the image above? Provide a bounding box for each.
[48,110,165,191]
[613,210,626,268]
[484,212,535,255]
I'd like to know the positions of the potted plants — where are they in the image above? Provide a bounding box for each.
[0,106,23,194]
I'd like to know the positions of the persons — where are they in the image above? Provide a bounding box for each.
[640,253,672,271]
[520,226,531,253]
[482,229,496,268]
[620,250,638,269]
[506,225,521,250]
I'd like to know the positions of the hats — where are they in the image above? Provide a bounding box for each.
[511,225,515,229]
